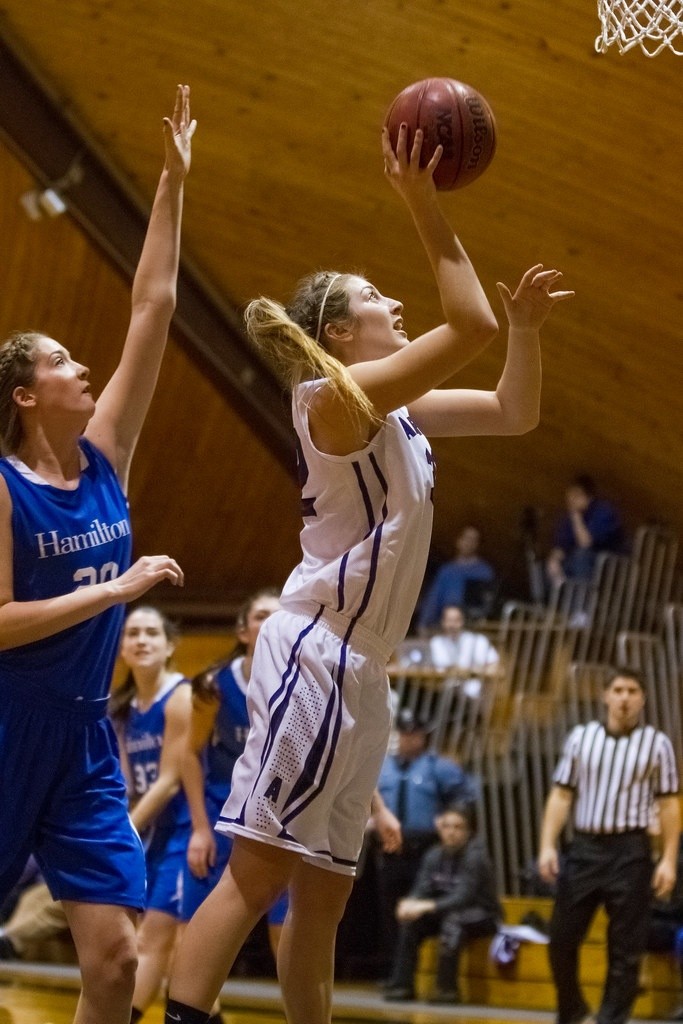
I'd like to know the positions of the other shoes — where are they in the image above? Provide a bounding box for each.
[558,1002,587,1024]
[387,988,414,1000]
[0,937,22,959]
[430,993,459,1005]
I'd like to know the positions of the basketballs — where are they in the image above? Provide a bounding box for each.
[382,76,501,194]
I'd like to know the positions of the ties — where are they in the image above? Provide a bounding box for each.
[398,766,407,823]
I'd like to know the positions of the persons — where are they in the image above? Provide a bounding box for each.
[0,476,683,1024]
[164,123,576,1024]
[0,85,197,1024]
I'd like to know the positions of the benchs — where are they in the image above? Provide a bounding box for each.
[415,896,683,1019]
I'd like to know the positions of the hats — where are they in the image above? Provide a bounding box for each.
[396,709,426,731]
